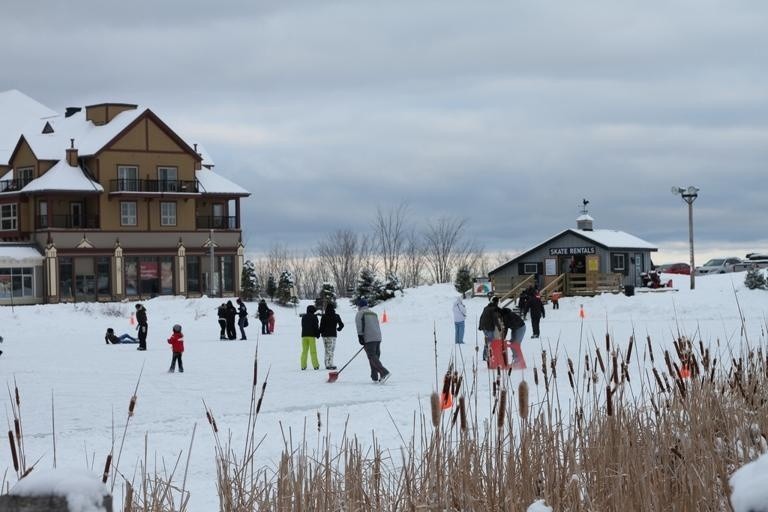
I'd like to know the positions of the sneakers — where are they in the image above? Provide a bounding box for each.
[378,369,388,381]
[326,366,335,369]
[530,334,538,338]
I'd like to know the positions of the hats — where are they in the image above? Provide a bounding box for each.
[357,299,367,307]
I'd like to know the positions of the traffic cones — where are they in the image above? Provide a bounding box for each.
[129,313,135,325]
[681,355,691,377]
[578,302,586,319]
[439,379,451,412]
[380,308,389,325]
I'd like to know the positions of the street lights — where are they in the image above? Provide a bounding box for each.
[668,185,698,289]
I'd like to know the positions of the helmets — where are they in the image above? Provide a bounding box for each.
[173,325,180,332]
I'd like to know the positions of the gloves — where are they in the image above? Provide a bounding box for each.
[358,335,364,345]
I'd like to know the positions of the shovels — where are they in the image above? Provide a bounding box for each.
[328,344,366,383]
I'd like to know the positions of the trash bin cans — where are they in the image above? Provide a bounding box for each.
[624,285,635,296]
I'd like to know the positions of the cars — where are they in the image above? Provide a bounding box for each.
[657,263,689,275]
[692,257,744,277]
[64,272,109,292]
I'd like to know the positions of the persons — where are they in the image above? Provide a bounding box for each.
[478,282,563,363]
[355,298,391,383]
[105,328,140,344]
[218,297,275,340]
[319,301,344,369]
[167,324,184,373]
[135,304,147,350]
[453,296,464,343]
[568,260,584,273]
[300,305,319,370]
